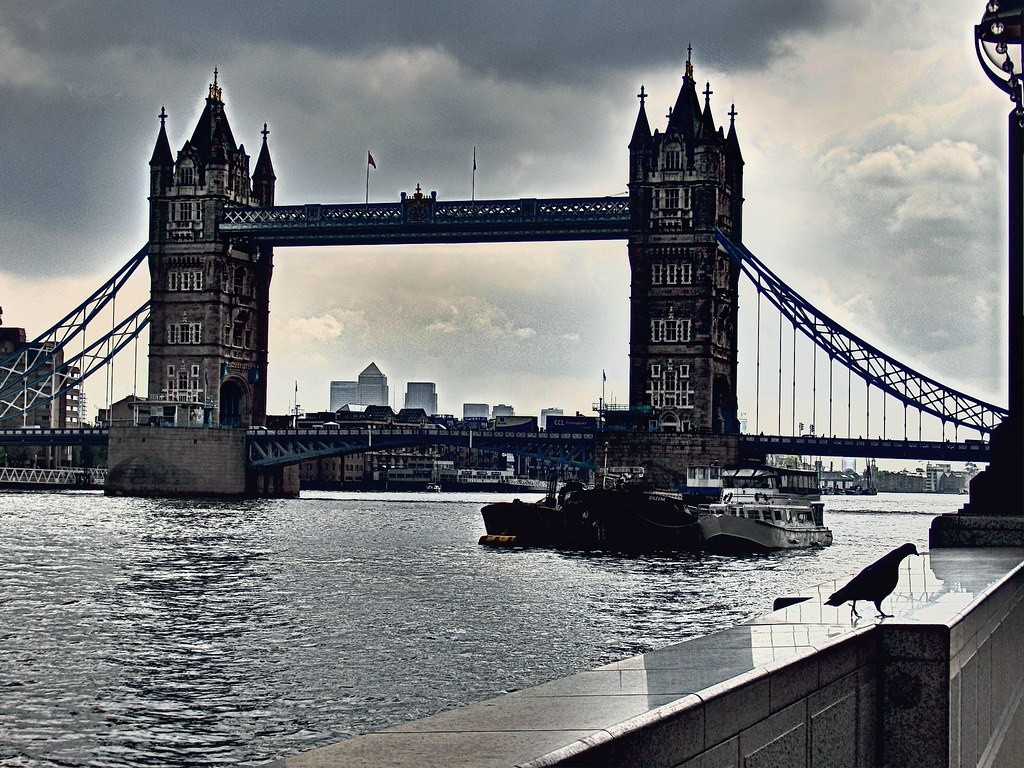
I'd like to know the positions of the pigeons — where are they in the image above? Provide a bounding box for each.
[822,542,920,620]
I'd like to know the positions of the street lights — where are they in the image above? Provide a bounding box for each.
[959,1,1024,514]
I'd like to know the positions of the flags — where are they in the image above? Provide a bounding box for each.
[603,369,607,382]
[368,154,377,169]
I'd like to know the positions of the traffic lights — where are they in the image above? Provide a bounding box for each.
[801,422,804,430]
[811,424,815,432]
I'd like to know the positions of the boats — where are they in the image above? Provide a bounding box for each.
[477,442,833,552]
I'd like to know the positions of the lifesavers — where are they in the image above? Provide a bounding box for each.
[754,491,768,505]
[724,491,733,504]
